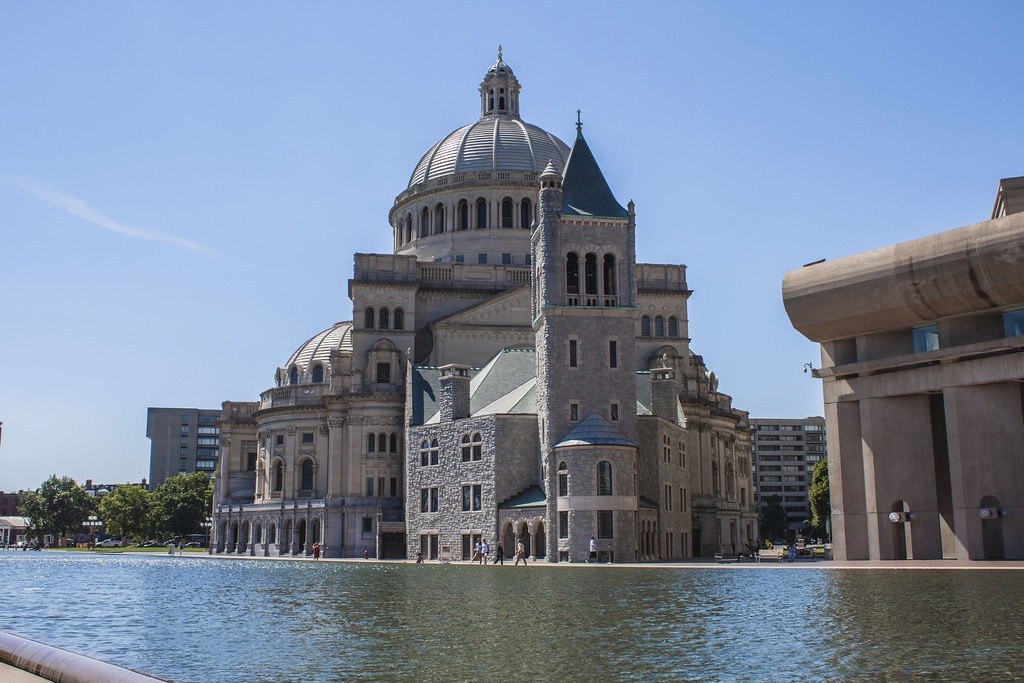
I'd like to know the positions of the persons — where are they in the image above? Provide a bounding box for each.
[787,543,797,562]
[514,539,527,565]
[736,537,762,563]
[178,542,184,556]
[472,538,489,564]
[493,541,504,565]
[585,537,600,564]
[312,540,320,560]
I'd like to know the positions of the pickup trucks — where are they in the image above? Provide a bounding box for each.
[95,539,122,548]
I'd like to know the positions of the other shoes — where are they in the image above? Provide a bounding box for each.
[606,562,612,565]
[585,560,588,563]
[595,561,597,564]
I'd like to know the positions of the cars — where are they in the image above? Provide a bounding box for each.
[136,539,202,549]
[772,539,787,546]
